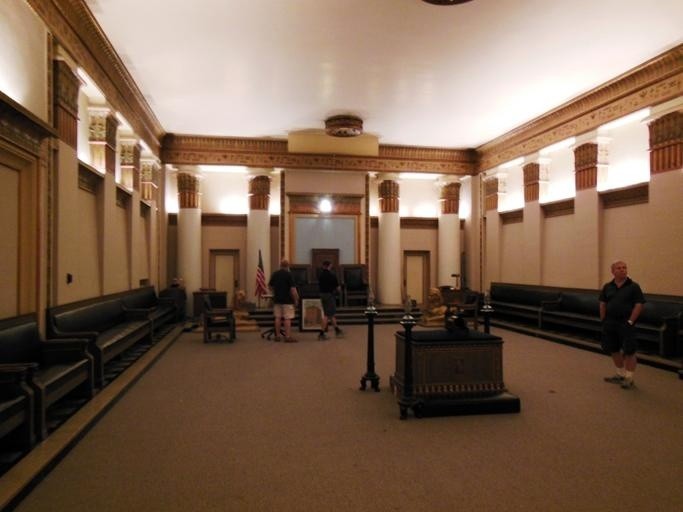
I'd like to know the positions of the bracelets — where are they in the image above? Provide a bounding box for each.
[628,318,633,327]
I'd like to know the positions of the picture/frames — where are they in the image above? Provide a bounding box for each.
[299,295,328,331]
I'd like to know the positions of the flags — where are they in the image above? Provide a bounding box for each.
[252,251,267,297]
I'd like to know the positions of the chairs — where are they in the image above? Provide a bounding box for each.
[340,263,369,306]
[201,293,235,343]
[431,287,485,329]
[287,262,307,299]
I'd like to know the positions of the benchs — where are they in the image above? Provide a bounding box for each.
[540,287,680,358]
[485,281,561,330]
[0,314,95,447]
[0,361,34,470]
[248,307,332,342]
[48,294,154,382]
[124,280,180,342]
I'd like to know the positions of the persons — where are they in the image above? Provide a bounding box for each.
[316,259,346,340]
[597,260,647,390]
[270,258,302,343]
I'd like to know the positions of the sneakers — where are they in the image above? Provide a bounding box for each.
[621,376,633,387]
[285,335,298,342]
[274,336,280,341]
[604,374,624,383]
